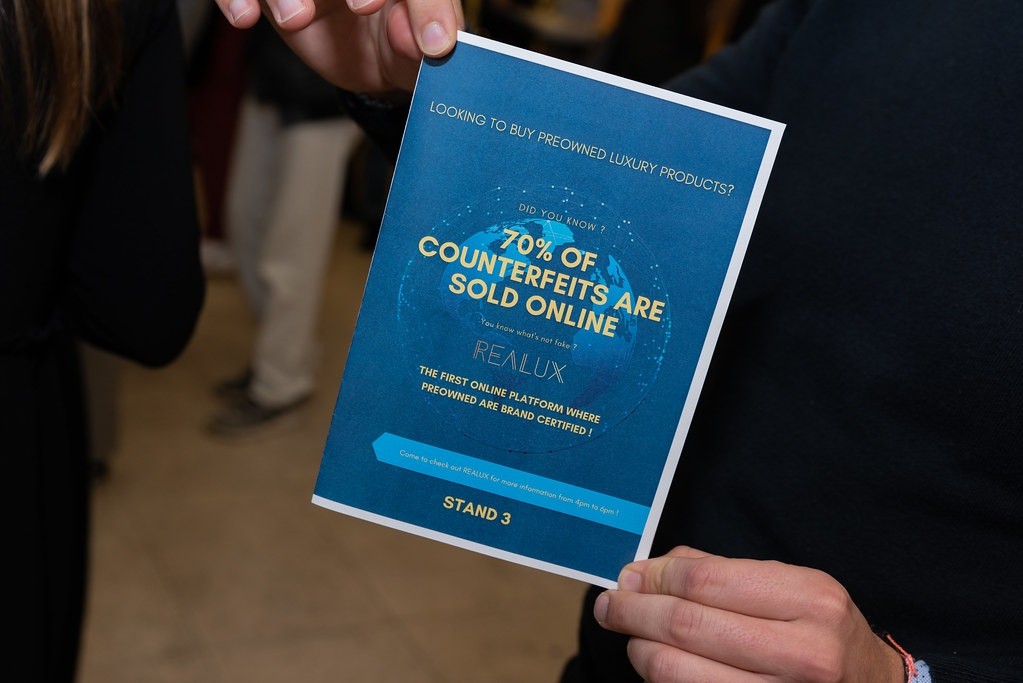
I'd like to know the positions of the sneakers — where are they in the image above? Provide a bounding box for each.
[203,370,317,443]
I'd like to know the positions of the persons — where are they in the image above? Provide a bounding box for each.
[223,15,364,411]
[1,0,208,682]
[220,1,1023,683]
[175,2,244,276]
